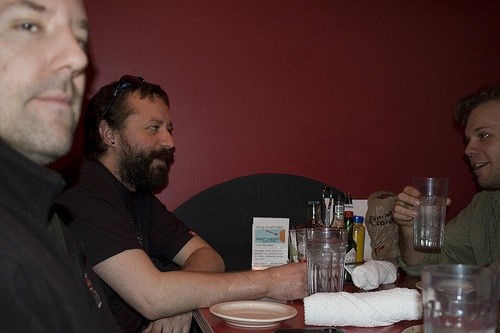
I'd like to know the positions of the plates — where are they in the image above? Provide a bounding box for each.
[402,324,423,333]
[415,280,476,295]
[209,301,298,327]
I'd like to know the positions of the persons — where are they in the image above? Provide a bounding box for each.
[391,84,500,301]
[0,0,124,333]
[53,74,310,333]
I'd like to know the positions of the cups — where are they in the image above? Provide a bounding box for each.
[307,242,346,298]
[412,177,449,255]
[421,264,498,333]
[289,230,297,262]
[298,228,307,262]
[305,228,349,284]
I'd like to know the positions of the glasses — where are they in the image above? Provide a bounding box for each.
[100,74,144,121]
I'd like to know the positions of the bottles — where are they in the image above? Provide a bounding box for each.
[307,184,365,262]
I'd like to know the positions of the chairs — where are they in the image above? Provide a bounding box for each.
[167,173,352,273]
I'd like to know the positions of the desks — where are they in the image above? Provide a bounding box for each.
[194,282,491,333]
